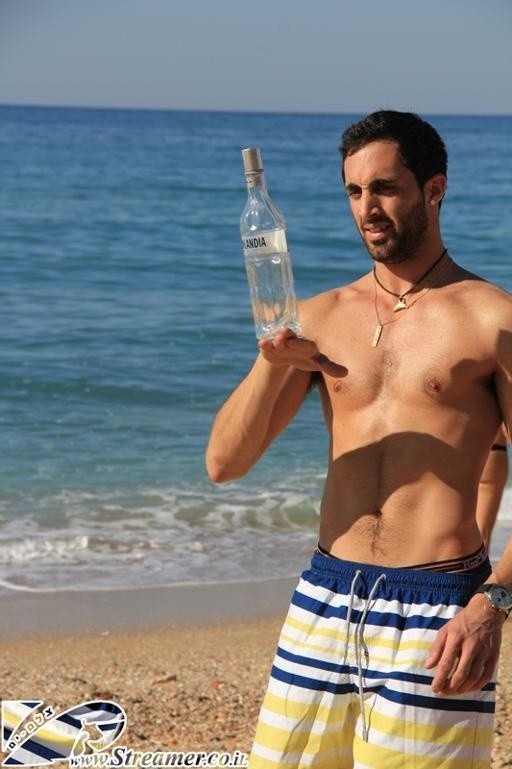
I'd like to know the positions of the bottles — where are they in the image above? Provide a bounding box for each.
[234,144,300,345]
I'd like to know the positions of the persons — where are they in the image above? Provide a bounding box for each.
[204,110,512,769]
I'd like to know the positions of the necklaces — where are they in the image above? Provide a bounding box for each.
[371,260,456,348]
[373,247,447,311]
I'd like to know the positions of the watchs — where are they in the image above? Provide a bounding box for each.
[473,583,512,619]
